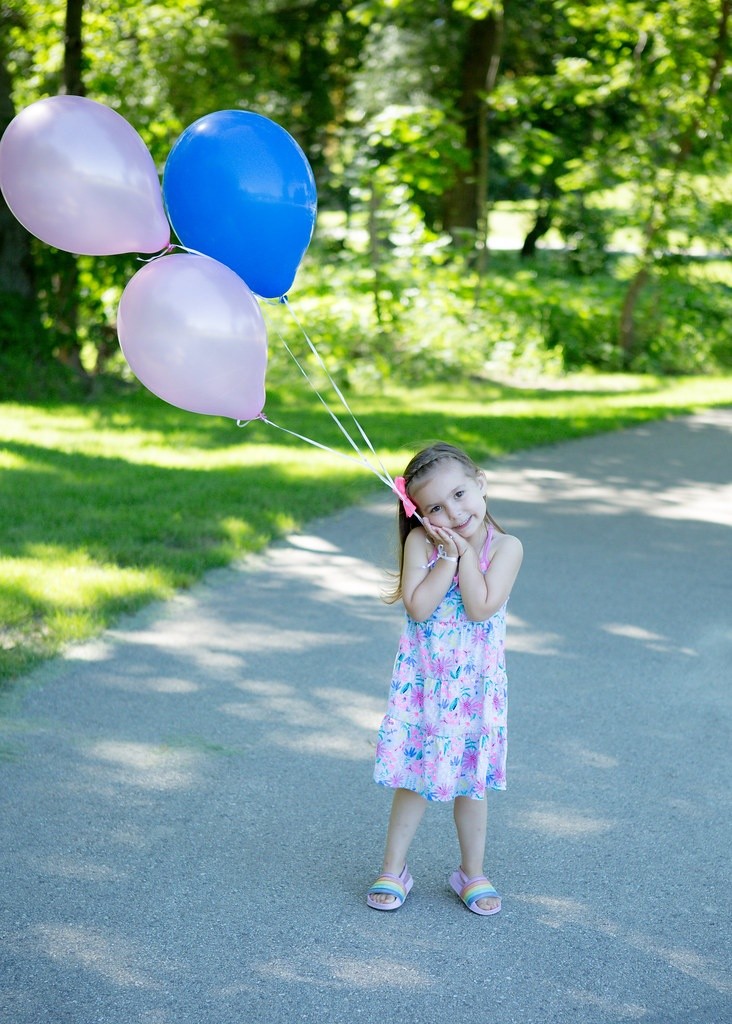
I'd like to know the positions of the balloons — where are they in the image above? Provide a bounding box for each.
[2,95,319,421]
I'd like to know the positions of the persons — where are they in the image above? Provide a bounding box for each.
[367,442,526,918]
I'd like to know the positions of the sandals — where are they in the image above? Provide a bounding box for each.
[449,865,503,916]
[367,860,414,912]
